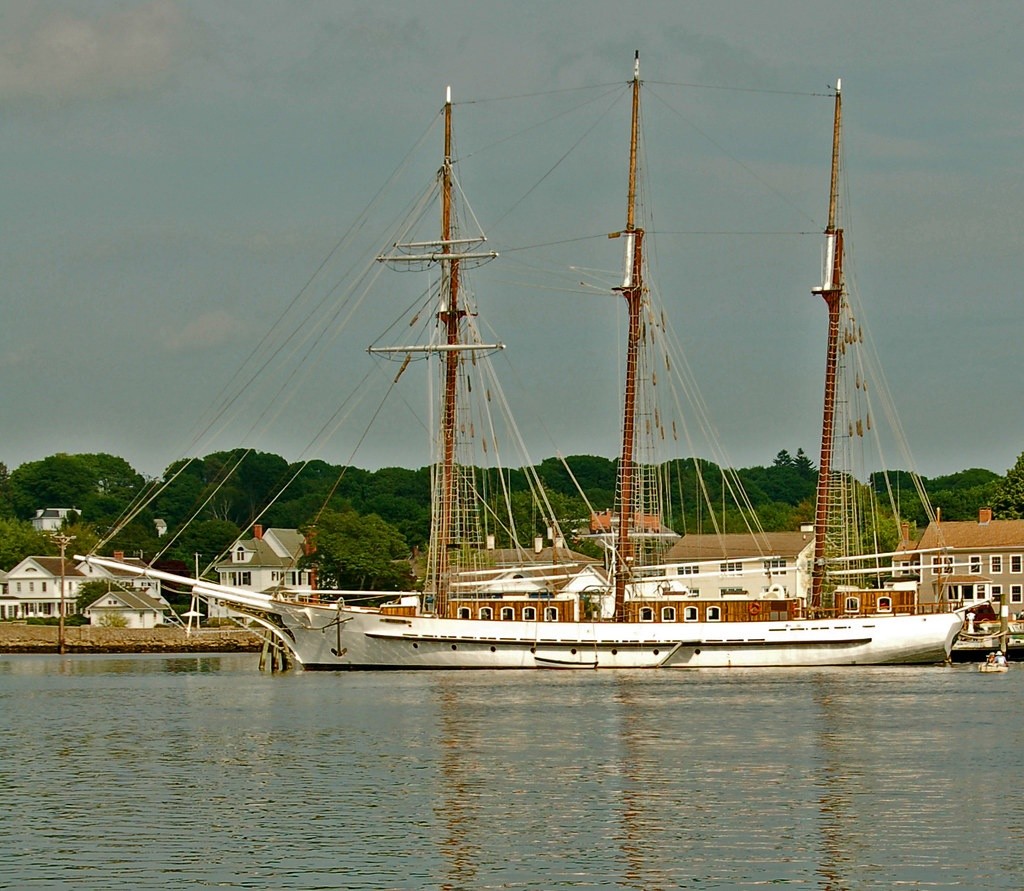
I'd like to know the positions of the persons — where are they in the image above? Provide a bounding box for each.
[966,610,975,634]
[321,595,333,604]
[988,651,1007,666]
[1017,609,1024,620]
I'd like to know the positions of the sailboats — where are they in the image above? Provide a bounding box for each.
[65,46,985,668]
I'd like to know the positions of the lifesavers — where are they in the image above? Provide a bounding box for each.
[749,602,760,614]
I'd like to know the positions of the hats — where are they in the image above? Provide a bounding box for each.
[995,651,1003,656]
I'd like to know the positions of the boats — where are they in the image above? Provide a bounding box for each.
[977,660,1010,673]
